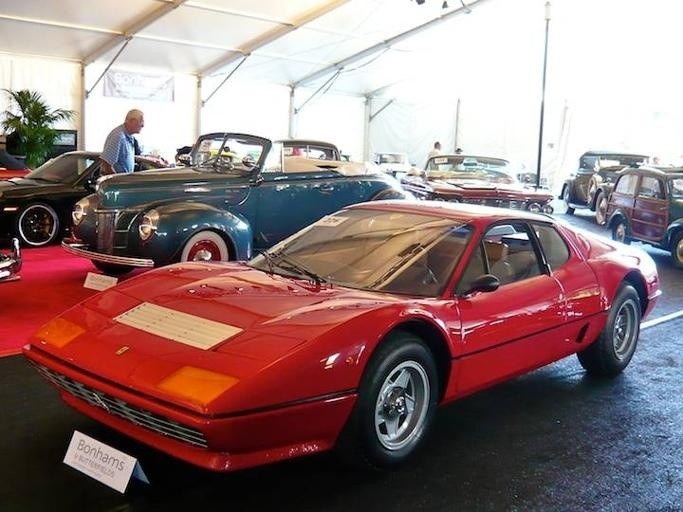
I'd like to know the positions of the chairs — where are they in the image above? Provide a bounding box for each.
[480,240,514,284]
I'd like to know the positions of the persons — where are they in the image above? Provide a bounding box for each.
[98,109,145,176]
[428,142,443,172]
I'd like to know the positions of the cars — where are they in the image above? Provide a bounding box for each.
[0,150,170,249]
[62,133,417,278]
[271,139,417,180]
[22,199,663,472]
[557,150,650,226]
[605,164,683,270]
[400,153,555,216]
